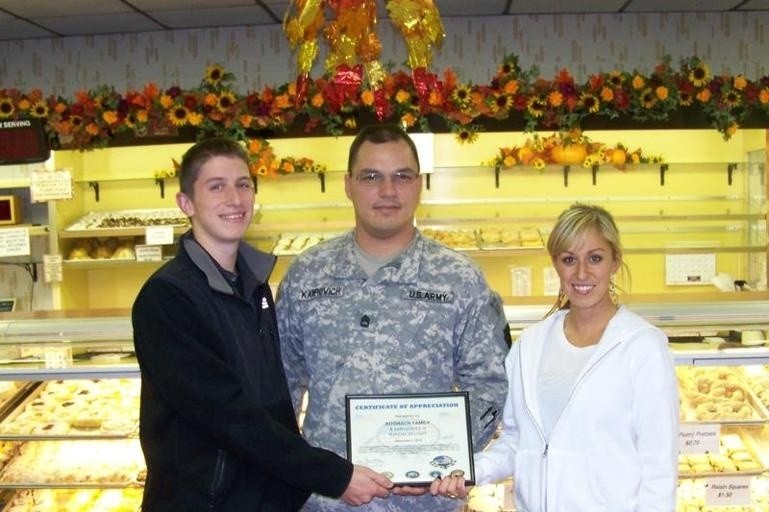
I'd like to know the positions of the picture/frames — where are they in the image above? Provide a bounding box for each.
[346,391,476,486]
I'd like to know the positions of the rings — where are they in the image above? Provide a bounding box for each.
[446,492,458,498]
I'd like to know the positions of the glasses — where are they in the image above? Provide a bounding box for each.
[357,173,416,185]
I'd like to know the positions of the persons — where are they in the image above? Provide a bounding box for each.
[273,125,513,512]
[132,140,395,512]
[430,202,679,512]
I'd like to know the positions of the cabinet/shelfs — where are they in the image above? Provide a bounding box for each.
[44,130,769,309]
[1,315,768,511]
